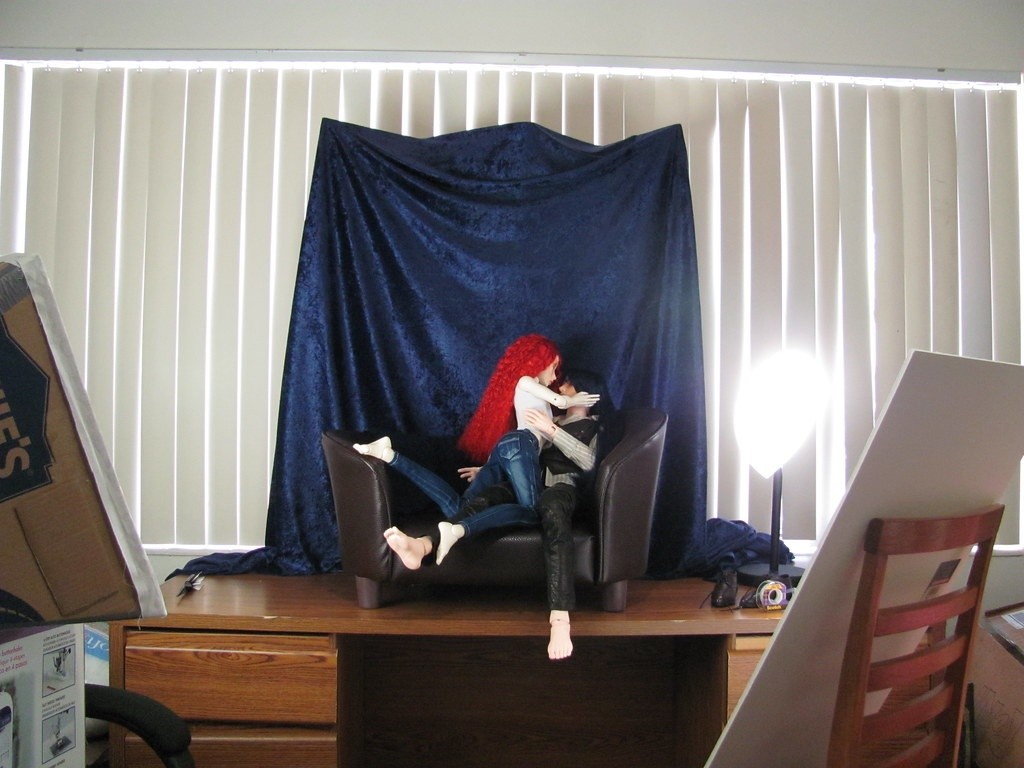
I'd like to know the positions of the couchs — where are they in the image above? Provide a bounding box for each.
[321,407,669,613]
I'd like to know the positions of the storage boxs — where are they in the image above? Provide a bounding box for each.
[0,250,171,637]
[0,623,86,768]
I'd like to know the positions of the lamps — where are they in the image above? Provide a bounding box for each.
[729,341,828,587]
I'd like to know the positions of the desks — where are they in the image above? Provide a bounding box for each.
[105,573,794,768]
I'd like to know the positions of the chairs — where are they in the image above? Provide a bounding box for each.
[813,501,1005,768]
[84,683,198,768]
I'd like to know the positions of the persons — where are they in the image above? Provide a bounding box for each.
[351,333,601,567]
[383,367,609,660]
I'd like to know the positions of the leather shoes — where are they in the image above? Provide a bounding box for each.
[720,574,792,611]
[699,571,738,609]
